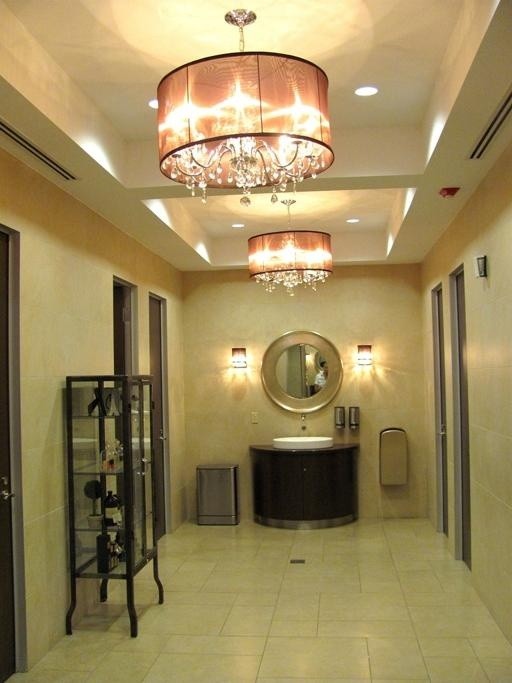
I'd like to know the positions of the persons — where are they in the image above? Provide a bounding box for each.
[314,359,328,391]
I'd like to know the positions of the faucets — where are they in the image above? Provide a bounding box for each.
[300,414,306,430]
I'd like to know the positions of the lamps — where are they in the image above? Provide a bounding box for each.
[477,255,487,276]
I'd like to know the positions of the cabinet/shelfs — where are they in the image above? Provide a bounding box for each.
[247,441,359,524]
[58,370,165,639]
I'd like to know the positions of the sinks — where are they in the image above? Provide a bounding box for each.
[272,436,333,449]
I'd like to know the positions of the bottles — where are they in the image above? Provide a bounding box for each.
[105,491,120,525]
[98,440,124,472]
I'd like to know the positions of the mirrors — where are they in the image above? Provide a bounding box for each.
[260,330,346,414]
